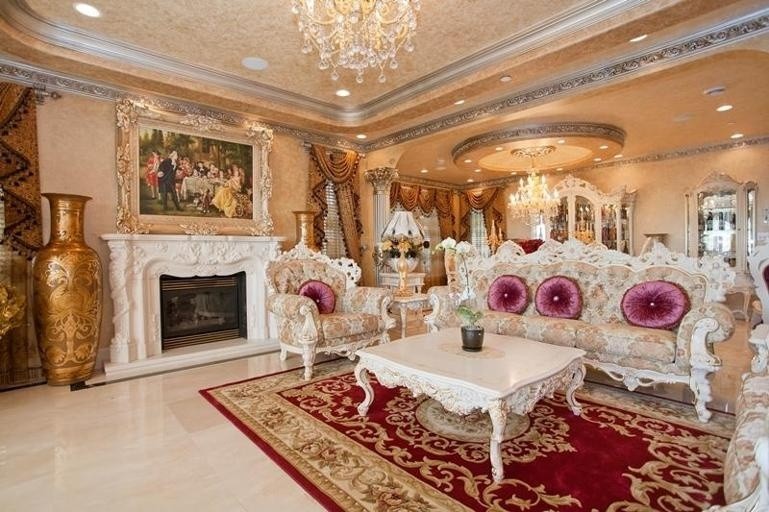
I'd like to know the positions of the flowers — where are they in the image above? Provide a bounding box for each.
[436,237,483,327]
[382,238,421,258]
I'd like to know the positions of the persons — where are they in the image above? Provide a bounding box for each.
[146,150,250,217]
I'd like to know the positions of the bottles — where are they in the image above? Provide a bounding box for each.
[549,199,630,254]
[698,192,737,260]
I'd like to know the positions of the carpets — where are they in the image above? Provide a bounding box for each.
[198,358,736,512]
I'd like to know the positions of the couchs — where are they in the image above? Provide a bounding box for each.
[423,239,735,424]
[706,240,769,512]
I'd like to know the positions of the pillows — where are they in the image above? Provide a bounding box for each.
[298,281,335,313]
[487,274,691,329]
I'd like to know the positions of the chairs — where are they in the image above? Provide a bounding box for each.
[265,240,395,381]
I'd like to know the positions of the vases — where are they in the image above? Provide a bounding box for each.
[31,192,104,386]
[291,210,320,254]
[388,257,419,273]
[461,326,483,348]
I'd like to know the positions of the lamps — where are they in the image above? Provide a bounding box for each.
[507,145,563,227]
[290,1,422,83]
[381,212,423,298]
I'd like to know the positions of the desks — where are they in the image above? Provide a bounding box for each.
[379,271,428,338]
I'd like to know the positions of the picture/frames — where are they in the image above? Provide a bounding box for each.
[115,98,273,236]
